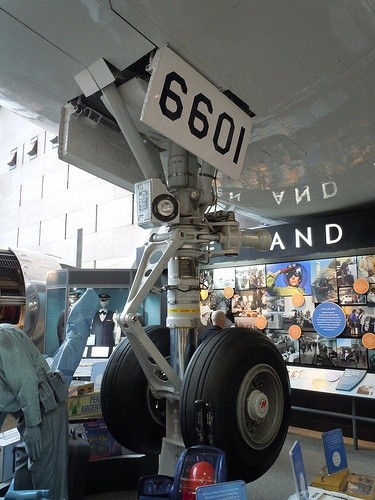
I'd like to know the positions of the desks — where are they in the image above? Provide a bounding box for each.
[288,486,364,500]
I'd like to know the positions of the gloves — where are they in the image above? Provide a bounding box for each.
[23,426,43,461]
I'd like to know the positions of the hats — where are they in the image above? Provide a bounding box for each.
[99,294,111,301]
[69,292,82,298]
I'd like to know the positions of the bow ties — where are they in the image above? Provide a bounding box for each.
[99,311,107,315]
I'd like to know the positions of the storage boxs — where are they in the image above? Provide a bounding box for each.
[310,465,349,493]
[346,473,375,500]
[0,380,122,485]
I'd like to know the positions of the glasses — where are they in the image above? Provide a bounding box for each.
[287,272,299,278]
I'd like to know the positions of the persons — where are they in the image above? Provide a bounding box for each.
[0,321,69,500]
[66,290,80,314]
[89,293,121,357]
[199,254,374,370]
